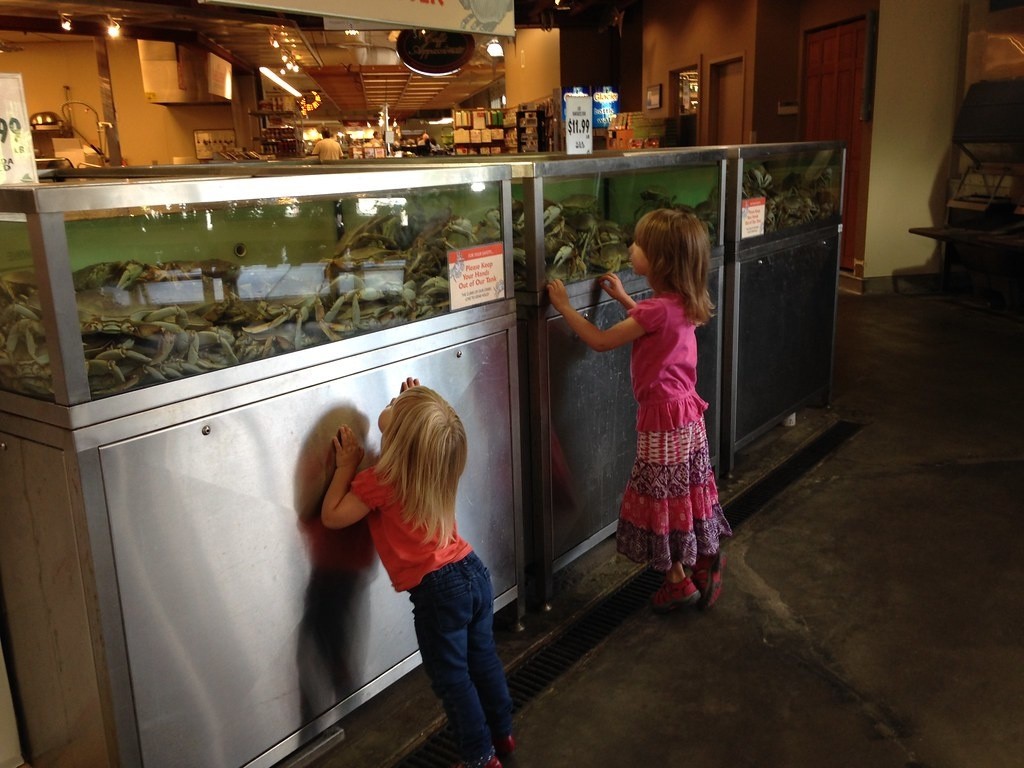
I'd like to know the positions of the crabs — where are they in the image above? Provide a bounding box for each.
[0,169,836,398]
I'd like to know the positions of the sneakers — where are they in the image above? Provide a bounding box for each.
[650,576,701,615]
[690,550,727,611]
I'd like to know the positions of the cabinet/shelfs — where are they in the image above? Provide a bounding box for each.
[250,110,305,158]
[0,138,854,768]
[449,87,558,156]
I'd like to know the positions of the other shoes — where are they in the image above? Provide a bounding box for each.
[492,735,516,760]
[448,755,503,768]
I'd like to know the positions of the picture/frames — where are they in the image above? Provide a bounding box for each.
[646,83,661,110]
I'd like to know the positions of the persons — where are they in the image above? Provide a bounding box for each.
[545,208,735,618]
[311,128,344,164]
[320,377,516,768]
[368,132,384,147]
